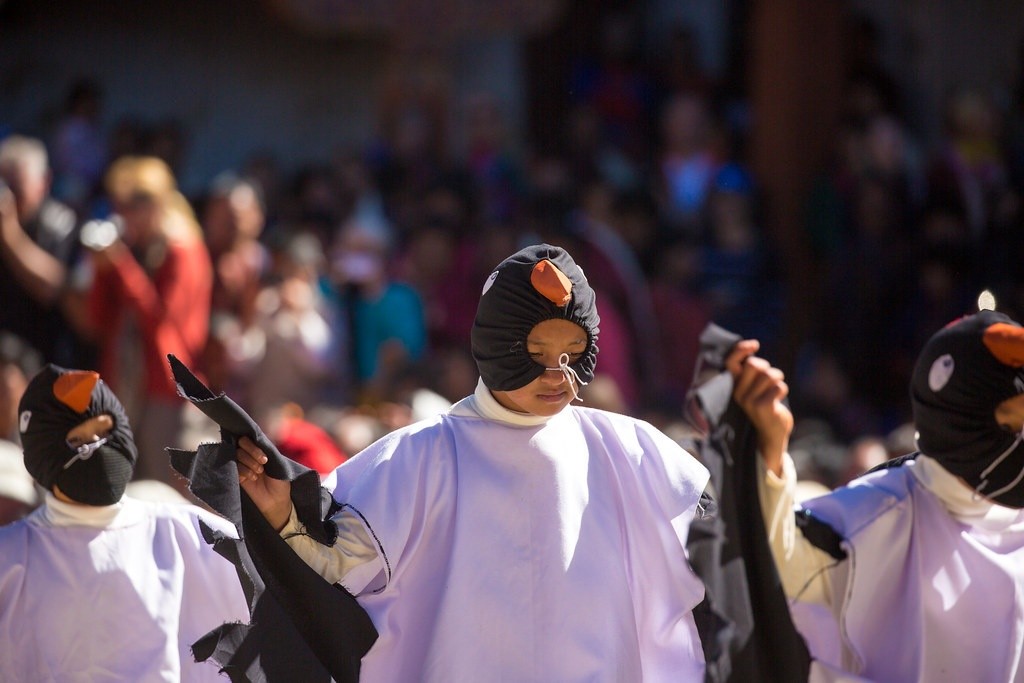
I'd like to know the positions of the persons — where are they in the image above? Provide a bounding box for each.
[236,243,718,683]
[0,66,1024,468]
[724,310,1024,683]
[0,362,251,683]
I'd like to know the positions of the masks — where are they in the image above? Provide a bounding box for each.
[55,441,133,505]
